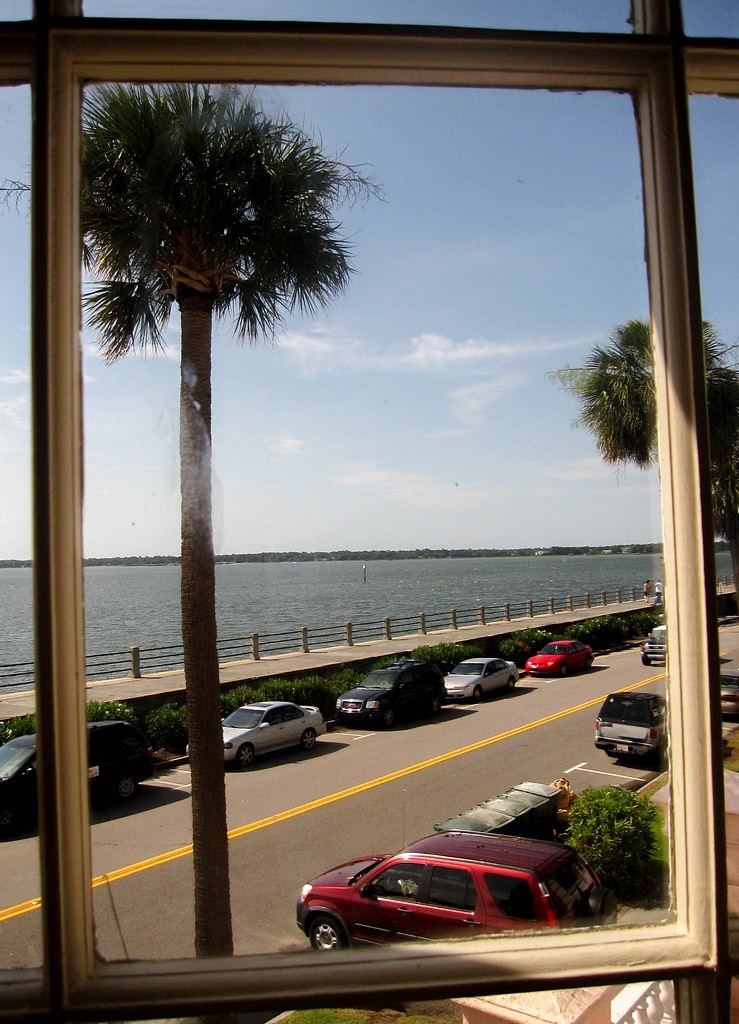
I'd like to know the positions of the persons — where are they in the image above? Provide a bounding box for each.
[654,580,663,596]
[654,593,662,605]
[642,580,652,603]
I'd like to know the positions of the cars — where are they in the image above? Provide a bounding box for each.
[186,701,327,770]
[719,668,739,718]
[442,658,519,701]
[525,641,595,678]
[0,720,156,830]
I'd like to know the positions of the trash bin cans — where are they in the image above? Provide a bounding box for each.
[433,782,561,835]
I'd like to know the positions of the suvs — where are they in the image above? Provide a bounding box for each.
[640,624,667,667]
[336,659,447,728]
[295,827,618,952]
[595,691,668,760]
